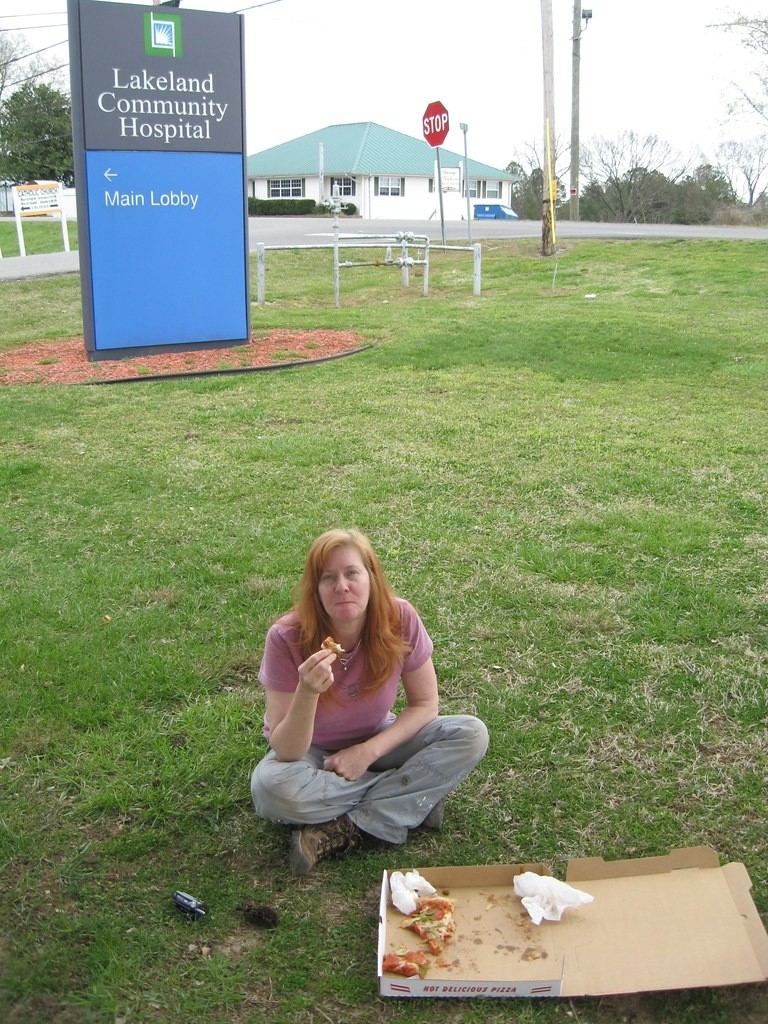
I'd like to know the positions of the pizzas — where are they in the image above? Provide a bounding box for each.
[321,636,345,652]
[382,948,431,980]
[400,896,457,955]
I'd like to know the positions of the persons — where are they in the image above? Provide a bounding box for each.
[249,529,489,875]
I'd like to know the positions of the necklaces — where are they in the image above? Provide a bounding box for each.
[337,638,361,671]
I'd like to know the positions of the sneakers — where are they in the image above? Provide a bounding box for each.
[424,799,444,831]
[289,814,364,876]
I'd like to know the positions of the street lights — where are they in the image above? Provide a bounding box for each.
[459,122,476,246]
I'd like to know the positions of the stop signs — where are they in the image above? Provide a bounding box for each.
[423,100,450,148]
